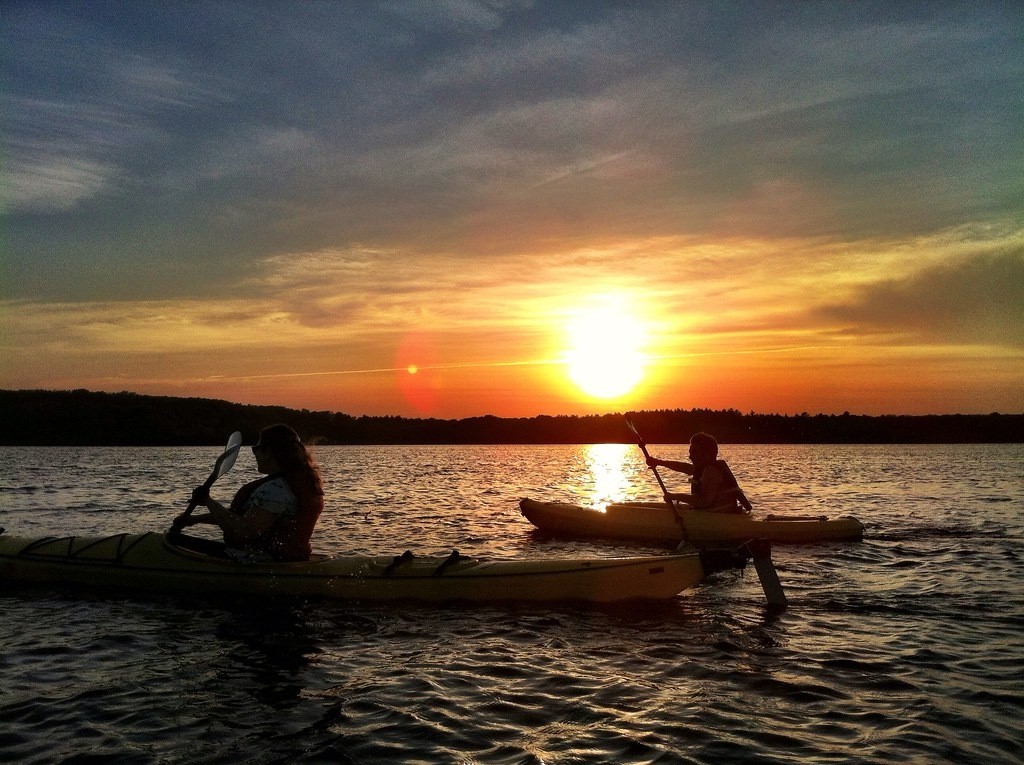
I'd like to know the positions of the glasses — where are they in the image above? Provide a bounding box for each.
[251,446,272,455]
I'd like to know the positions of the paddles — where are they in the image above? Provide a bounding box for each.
[625,417,693,553]
[173,430,240,535]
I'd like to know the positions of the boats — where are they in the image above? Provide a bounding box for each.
[517,497,865,544]
[2,528,735,615]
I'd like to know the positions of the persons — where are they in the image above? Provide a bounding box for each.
[173,423,324,561]
[646,433,738,512]
